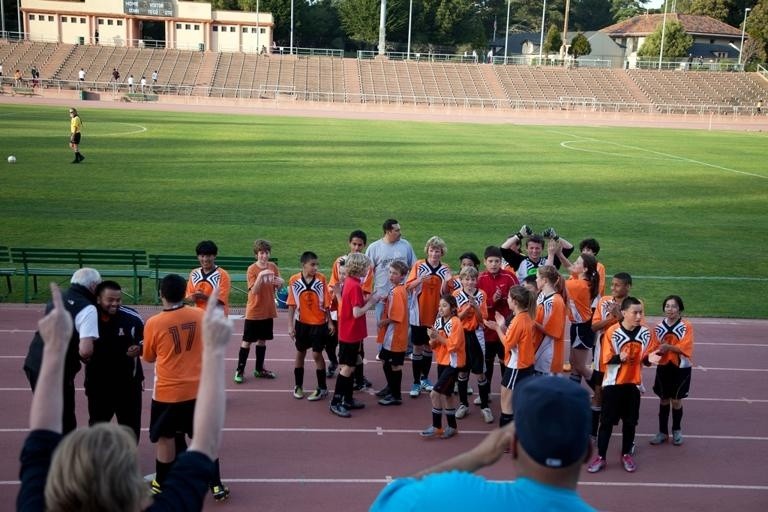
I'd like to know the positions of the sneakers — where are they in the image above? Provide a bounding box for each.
[211,486,228,502]
[253,368,275,379]
[587,457,607,473]
[420,425,457,438]
[672,428,682,445]
[622,454,636,472]
[71,156,84,163]
[234,370,243,382]
[294,362,371,416]
[650,432,668,445]
[377,379,435,405]
[145,480,161,498]
[455,379,494,424]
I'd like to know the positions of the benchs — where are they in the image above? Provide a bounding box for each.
[0,244,279,304]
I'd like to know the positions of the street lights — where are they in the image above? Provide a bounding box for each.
[738,7,751,64]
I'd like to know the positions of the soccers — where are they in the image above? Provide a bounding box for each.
[6,156,16,164]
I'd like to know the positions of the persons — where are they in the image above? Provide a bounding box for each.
[259,44,267,54]
[14,69,22,88]
[464,45,493,65]
[152,70,159,85]
[32,69,39,88]
[32,66,36,82]
[237,221,696,471]
[112,68,120,87]
[0,63,3,85]
[79,68,86,90]
[127,75,135,93]
[373,375,600,512]
[141,76,147,93]
[17,241,233,511]
[69,107,85,163]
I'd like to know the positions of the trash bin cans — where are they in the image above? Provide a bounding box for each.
[80,37,84,46]
[80,91,87,100]
[199,44,204,52]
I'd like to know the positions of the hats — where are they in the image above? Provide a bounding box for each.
[512,375,590,468]
